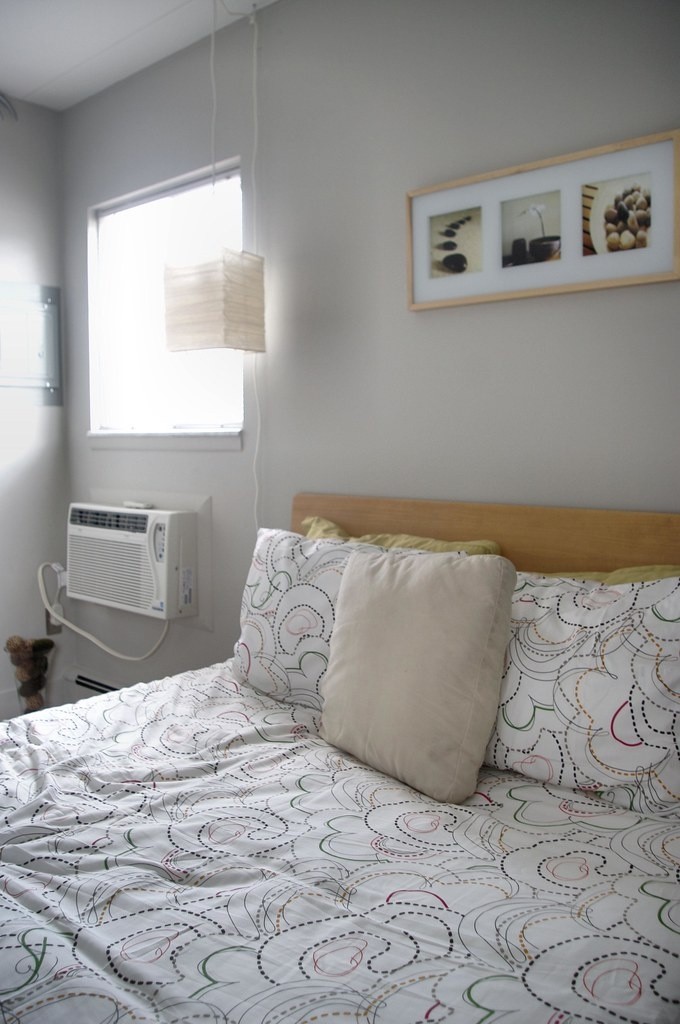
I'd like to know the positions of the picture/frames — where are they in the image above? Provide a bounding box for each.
[404,128,680,311]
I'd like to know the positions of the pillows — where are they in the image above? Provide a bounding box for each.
[315,543,518,805]
[519,566,678,587]
[230,527,357,711]
[480,572,680,817]
[298,515,502,556]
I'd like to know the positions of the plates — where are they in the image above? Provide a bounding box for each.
[590,173,651,254]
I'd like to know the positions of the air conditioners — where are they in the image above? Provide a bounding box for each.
[66,499,199,620]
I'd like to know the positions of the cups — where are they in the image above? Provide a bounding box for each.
[513,238,527,265]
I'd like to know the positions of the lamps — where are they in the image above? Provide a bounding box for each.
[162,249,266,354]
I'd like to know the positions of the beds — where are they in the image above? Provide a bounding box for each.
[0,491,680,1024]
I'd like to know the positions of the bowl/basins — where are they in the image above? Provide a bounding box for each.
[529,235,561,258]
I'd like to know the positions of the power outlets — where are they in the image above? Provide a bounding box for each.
[45,607,63,636]
